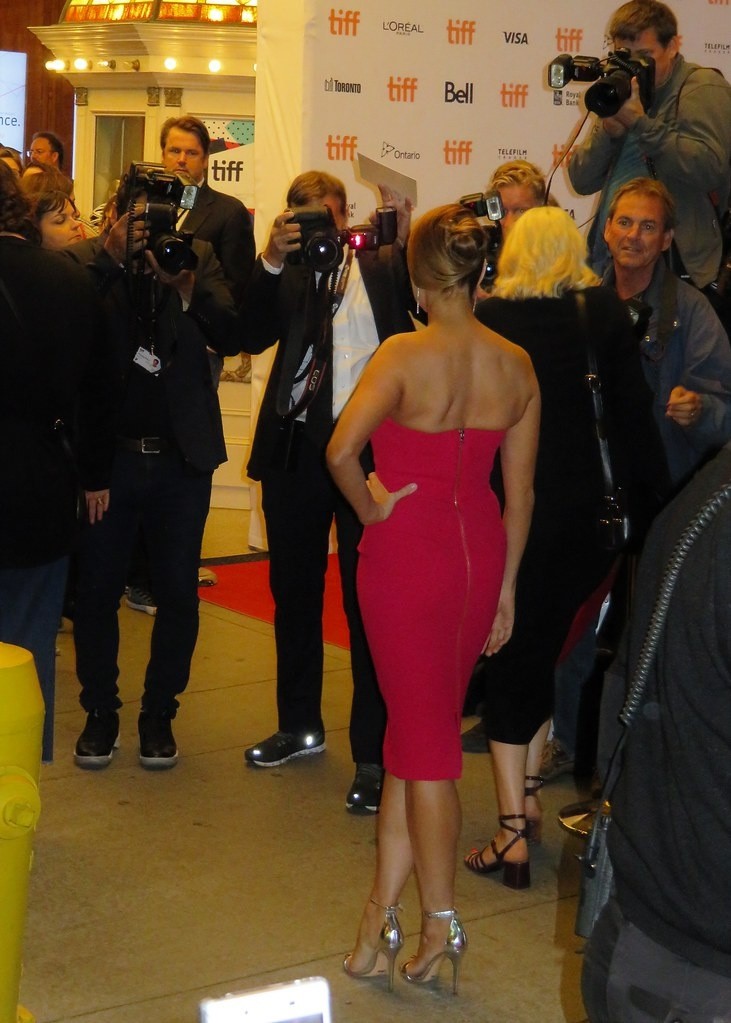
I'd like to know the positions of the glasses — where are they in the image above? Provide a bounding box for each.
[27,149,44,158]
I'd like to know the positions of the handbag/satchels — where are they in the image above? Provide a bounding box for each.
[594,489,629,551]
[573,816,615,939]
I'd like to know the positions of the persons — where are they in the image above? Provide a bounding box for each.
[464,207,664,889]
[486,161,545,238]
[325,202,541,992]
[0,128,78,762]
[579,439,730,1023]
[158,116,250,584]
[598,177,731,505]
[240,172,429,813]
[56,172,240,768]
[566,0,730,333]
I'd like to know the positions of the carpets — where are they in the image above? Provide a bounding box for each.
[198,553,351,652]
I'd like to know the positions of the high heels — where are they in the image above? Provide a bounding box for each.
[343,897,404,993]
[399,906,469,995]
[525,775,548,843]
[465,813,531,889]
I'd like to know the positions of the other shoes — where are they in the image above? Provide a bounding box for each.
[538,741,574,776]
[462,723,492,753]
[198,568,218,586]
[126,588,158,616]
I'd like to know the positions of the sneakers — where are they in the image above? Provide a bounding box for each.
[244,729,325,766]
[345,762,383,811]
[74,712,121,766]
[138,711,180,768]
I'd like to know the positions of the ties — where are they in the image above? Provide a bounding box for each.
[302,271,332,463]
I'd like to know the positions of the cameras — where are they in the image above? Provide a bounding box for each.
[458,190,506,252]
[126,159,199,276]
[284,205,398,271]
[547,47,656,118]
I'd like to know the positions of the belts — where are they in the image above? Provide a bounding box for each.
[120,435,172,454]
